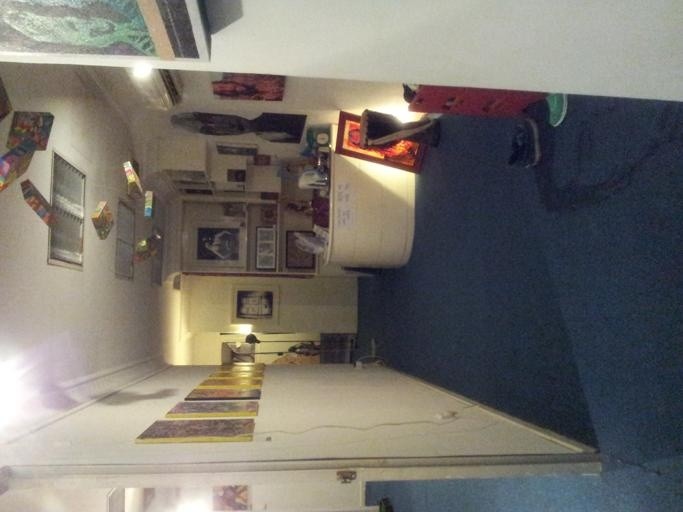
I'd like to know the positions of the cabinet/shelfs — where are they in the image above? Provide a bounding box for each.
[155,138,282,203]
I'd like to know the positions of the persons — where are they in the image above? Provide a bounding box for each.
[171,112,303,143]
[196,230,241,260]
[211,75,283,100]
[286,197,330,227]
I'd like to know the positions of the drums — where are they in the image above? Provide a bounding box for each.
[358,110,440,149]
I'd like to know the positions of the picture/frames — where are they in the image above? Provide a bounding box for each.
[186,217,317,273]
[229,279,283,326]
[0,1,211,64]
[333,110,427,174]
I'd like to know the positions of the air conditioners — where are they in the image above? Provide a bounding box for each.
[124,68,185,110]
